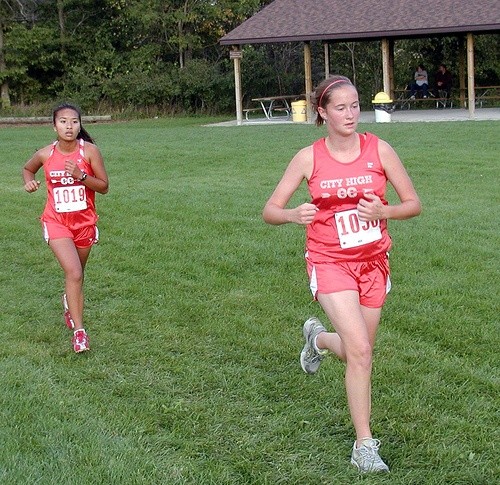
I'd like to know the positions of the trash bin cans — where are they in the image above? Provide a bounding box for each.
[372,92,394,123]
[291,99,306,122]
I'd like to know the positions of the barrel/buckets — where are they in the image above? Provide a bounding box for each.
[290,99,307,122]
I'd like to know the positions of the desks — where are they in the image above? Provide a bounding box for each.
[476,86,500,95]
[252,94,298,121]
[395,89,449,109]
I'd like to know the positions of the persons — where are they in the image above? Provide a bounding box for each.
[432,64,452,100]
[263,75,422,472]
[408,64,428,99]
[22,105,109,352]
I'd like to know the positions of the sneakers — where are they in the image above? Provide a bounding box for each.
[72,329,89,353]
[300,317,326,374]
[62,295,77,329]
[350,440,389,474]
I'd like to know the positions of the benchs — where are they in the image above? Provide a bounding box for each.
[394,97,458,110]
[476,95,500,105]
[242,105,289,122]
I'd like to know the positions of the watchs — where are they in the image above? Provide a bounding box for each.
[81,171,87,181]
